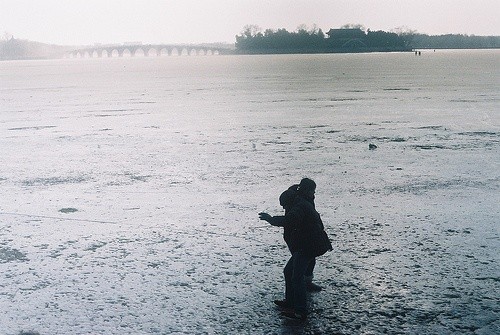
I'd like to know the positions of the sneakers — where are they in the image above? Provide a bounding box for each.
[274,299,294,308]
[281,312,300,320]
[304,283,322,291]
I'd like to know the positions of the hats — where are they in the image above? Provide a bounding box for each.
[279,190,298,205]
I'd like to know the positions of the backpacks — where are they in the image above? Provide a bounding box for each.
[300,210,328,257]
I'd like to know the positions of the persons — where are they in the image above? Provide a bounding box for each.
[257,185,334,322]
[291,177,324,291]
